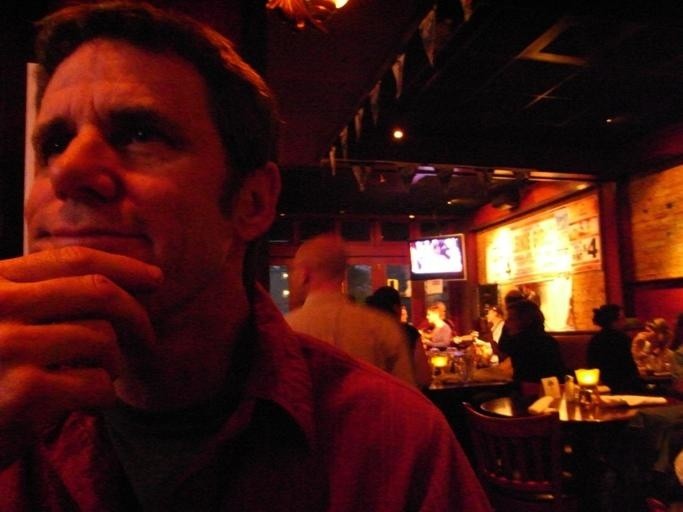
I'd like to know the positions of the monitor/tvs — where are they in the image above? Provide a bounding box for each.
[408,233,469,282]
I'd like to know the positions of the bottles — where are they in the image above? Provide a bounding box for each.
[564,375,574,402]
[424,347,466,379]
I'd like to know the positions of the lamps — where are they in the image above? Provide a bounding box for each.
[575,368,602,402]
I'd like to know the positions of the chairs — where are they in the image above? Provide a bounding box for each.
[462,401,580,512]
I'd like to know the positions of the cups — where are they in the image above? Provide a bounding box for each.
[578,385,599,408]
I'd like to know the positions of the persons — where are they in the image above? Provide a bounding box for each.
[1,0,496,511]
[343,261,374,304]
[399,304,408,322]
[420,301,456,337]
[361,285,433,389]
[474,288,681,391]
[416,303,453,349]
[277,231,415,388]
